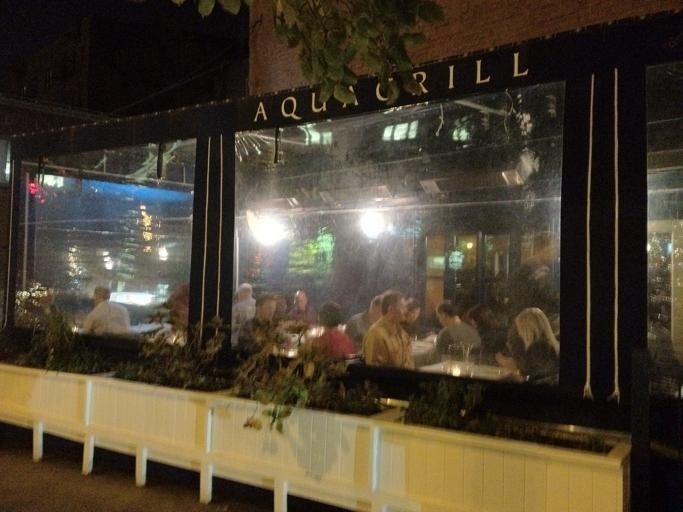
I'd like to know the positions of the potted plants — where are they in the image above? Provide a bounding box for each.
[2,284,212,504]
[212,321,634,510]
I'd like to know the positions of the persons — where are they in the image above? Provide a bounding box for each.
[647,301,683,397]
